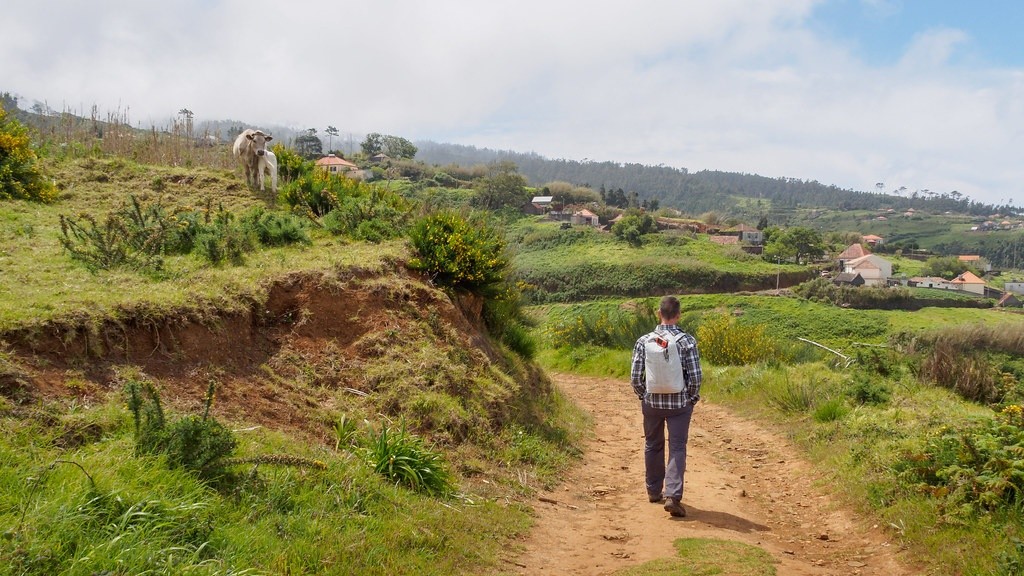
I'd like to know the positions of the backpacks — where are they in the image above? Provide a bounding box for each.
[644,332,688,394]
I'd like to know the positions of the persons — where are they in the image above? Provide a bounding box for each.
[631,296,702,517]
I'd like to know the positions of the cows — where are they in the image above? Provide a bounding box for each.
[232,128,274,190]
[257,149,277,192]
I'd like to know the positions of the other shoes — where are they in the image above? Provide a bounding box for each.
[649,493,663,502]
[664,498,686,517]
